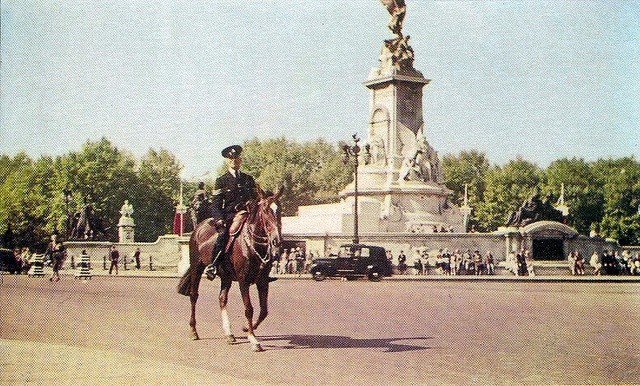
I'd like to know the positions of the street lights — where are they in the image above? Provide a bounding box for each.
[342,131,371,244]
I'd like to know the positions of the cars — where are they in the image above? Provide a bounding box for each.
[308,242,391,282]
[0,249,21,276]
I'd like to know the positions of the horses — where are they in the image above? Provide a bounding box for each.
[175,183,286,354]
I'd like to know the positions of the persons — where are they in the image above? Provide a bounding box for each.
[133,248,141,270]
[34,251,43,278]
[108,245,120,274]
[194,193,208,225]
[270,247,332,274]
[568,248,640,276]
[382,248,535,277]
[192,181,207,204]
[12,246,32,275]
[211,145,265,276]
[42,234,63,282]
[78,246,92,279]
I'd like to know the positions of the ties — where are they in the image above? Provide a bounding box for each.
[235,169,239,177]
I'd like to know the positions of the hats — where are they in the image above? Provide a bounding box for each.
[222,145,242,157]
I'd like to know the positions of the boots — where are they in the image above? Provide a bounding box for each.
[206,241,225,279]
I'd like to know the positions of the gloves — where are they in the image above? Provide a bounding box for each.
[215,220,227,233]
